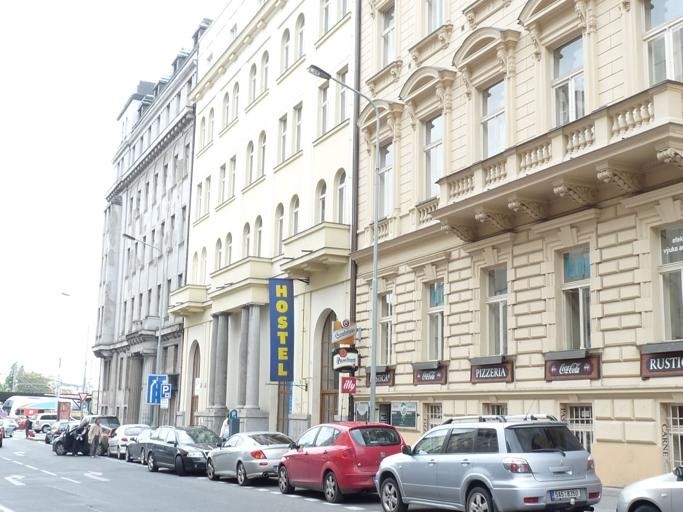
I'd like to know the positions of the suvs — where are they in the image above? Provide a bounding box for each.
[372,412,603,512]
[275,421,410,504]
[143,424,225,478]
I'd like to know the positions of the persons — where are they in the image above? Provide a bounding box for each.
[87,418,102,458]
[22,415,31,438]
[223,419,230,438]
[218,410,229,437]
[71,422,89,455]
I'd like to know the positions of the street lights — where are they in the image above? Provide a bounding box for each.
[60,291,93,393]
[122,234,166,431]
[303,61,385,423]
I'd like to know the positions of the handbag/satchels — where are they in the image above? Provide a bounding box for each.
[28,430,35,438]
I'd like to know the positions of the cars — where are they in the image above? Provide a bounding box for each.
[204,429,297,486]
[1,395,157,466]
[613,462,682,512]
